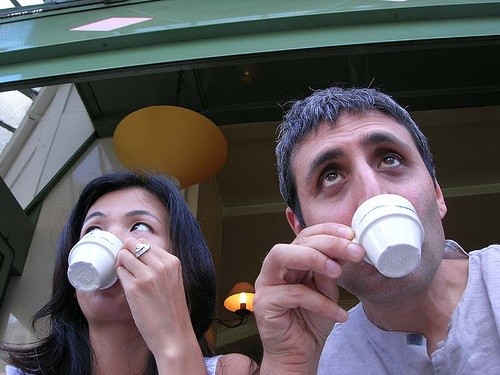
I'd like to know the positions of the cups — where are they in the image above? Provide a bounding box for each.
[350,193,424,278]
[67,230,124,291]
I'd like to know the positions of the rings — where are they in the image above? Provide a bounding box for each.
[133,243,152,258]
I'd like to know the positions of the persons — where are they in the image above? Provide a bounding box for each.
[253,85,499,374]
[0,167,260,375]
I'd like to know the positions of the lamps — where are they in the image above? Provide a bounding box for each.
[210,282,255,328]
[112,70,228,191]
